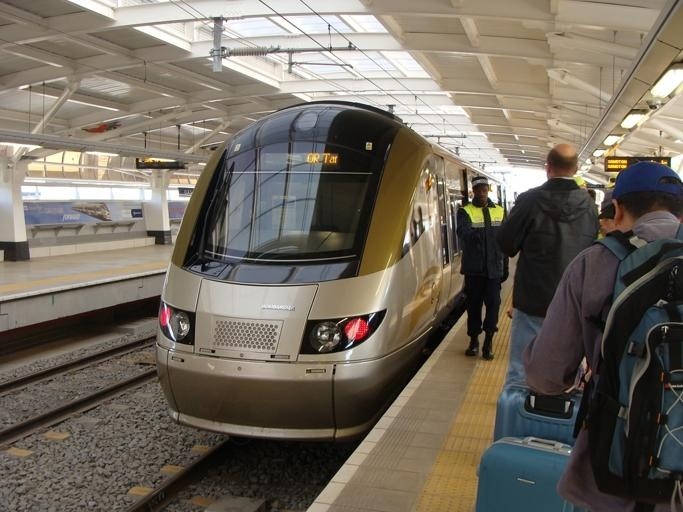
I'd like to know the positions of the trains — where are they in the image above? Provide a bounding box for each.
[68,202,110,221]
[147,95,518,444]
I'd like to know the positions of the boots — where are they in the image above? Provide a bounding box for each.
[482,332,494,360]
[465,335,479,356]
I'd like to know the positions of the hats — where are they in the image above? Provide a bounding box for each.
[597,161,683,219]
[472,176,488,187]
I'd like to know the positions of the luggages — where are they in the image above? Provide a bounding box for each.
[475,435,583,512]
[493,380,581,447]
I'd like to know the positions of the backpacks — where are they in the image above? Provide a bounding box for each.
[591,221,683,504]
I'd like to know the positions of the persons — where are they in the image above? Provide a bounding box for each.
[596,190,616,239]
[455,177,510,361]
[522,161,683,511]
[588,188,602,240]
[497,143,600,382]
[571,175,586,192]
